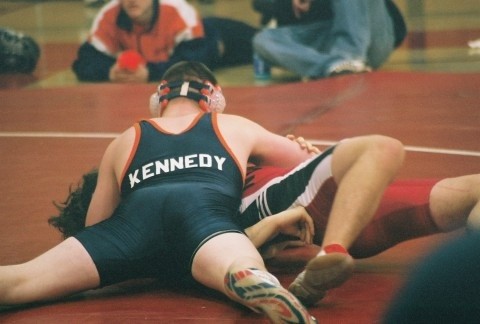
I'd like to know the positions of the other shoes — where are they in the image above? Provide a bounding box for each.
[286,244,356,308]
[327,60,372,74]
[271,66,292,78]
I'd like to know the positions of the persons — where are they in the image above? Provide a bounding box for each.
[251,0,408,82]
[0,59,318,324]
[71,0,260,83]
[383,230,480,324]
[47,132,480,307]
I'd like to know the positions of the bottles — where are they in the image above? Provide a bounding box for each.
[253,53,271,80]
